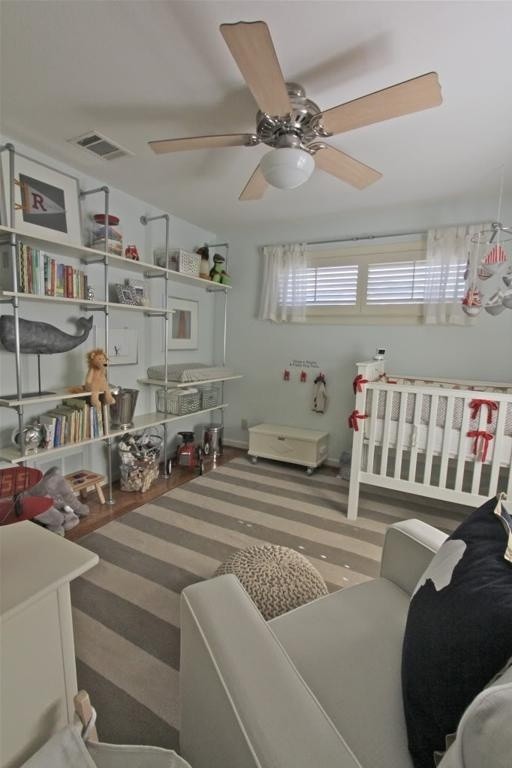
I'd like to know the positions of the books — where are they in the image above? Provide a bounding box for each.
[0,235,89,300]
[38,398,106,451]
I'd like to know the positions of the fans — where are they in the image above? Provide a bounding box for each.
[144,18,445,202]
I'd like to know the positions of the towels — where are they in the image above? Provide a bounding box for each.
[311,373,328,418]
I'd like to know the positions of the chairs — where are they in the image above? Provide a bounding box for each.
[176,516,512,766]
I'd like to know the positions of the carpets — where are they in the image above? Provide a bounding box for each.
[70,454,474,753]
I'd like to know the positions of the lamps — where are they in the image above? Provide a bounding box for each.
[257,132,316,189]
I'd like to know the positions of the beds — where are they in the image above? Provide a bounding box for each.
[345,361,512,519]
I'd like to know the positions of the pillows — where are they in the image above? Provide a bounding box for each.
[401,491,512,766]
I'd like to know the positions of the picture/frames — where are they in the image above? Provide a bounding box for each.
[93,324,139,365]
[125,279,148,304]
[115,283,138,305]
[0,144,84,249]
[168,296,200,351]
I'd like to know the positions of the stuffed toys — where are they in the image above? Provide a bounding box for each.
[209,254,230,284]
[65,348,116,408]
[15,466,90,538]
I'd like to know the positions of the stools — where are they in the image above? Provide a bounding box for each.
[207,542,329,621]
[63,468,107,507]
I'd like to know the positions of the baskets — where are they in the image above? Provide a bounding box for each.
[197,386,219,409]
[153,249,201,277]
[156,389,201,415]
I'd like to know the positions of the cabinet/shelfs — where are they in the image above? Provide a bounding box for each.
[246,423,330,474]
[0,225,245,461]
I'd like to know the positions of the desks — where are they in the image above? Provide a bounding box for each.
[1,515,101,767]
